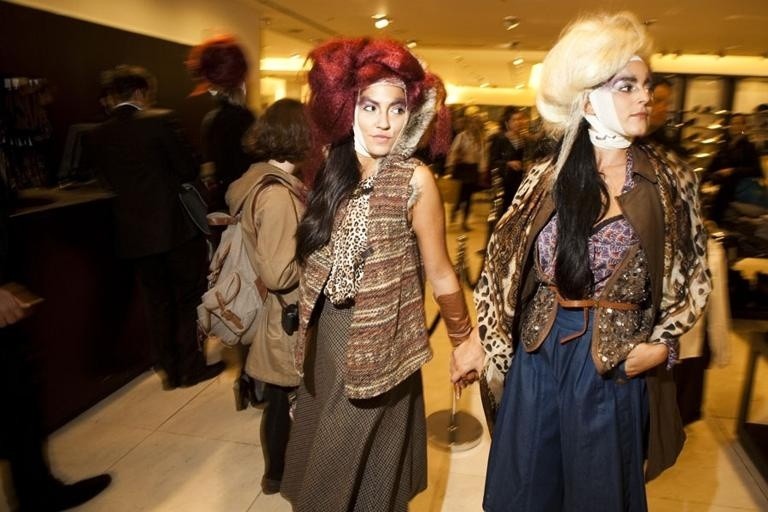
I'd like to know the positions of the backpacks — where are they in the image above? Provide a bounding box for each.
[197,178,268,346]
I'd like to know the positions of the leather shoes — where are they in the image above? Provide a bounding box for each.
[169,360,226,389]
[18,474,112,512]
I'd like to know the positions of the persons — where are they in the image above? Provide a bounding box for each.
[450,12,714,512]
[280,36,478,512]
